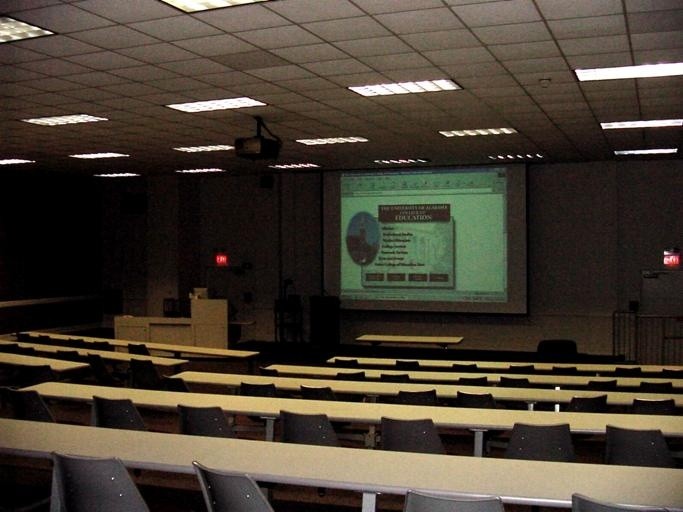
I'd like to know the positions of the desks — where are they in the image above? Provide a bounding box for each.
[114,288,255,349]
[1,419,683,512]
[17,382,683,456]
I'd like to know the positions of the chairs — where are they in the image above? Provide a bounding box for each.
[604,425,674,467]
[507,424,579,463]
[280,410,342,448]
[2,386,55,422]
[177,404,237,439]
[192,461,275,512]
[93,396,147,431]
[404,489,505,511]
[572,493,672,511]
[380,417,448,454]
[51,452,151,511]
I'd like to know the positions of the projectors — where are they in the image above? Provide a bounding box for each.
[236,137,281,162]
